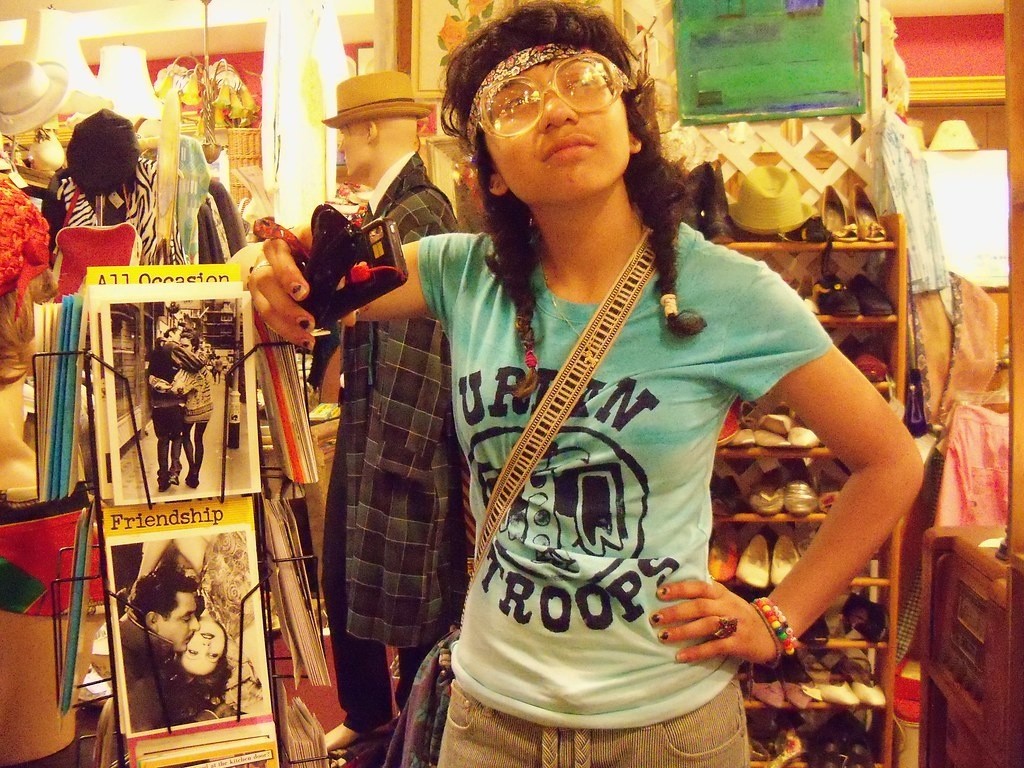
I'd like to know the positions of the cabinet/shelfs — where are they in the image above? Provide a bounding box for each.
[706,213,910,768]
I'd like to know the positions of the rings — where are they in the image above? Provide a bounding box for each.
[711,615,740,637]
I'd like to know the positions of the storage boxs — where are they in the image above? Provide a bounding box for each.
[227,127,262,211]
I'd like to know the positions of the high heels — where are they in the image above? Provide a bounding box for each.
[822,181,887,242]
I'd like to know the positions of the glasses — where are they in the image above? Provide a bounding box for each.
[475,53,633,141]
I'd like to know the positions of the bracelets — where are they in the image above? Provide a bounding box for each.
[752,595,802,671]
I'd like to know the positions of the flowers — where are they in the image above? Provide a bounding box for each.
[437,0,495,67]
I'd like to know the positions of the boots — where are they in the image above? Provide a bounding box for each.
[694,161,734,243]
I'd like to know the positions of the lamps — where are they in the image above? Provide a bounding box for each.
[153,0,260,165]
[66,42,165,138]
[926,117,980,152]
[20,3,117,177]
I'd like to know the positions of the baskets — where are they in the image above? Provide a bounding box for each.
[226,128,263,208]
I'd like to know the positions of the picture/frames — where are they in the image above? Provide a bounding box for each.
[411,0,626,100]
[425,137,492,236]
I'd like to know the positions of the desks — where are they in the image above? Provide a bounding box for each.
[919,520,1022,768]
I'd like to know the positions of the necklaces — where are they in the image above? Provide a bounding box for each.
[536,210,638,368]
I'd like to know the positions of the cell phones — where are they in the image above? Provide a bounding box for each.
[291,218,408,329]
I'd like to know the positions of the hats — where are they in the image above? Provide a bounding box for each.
[728,164,817,234]
[0,59,70,135]
[322,71,432,129]
[65,110,139,198]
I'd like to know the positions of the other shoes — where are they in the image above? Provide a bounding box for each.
[814,269,894,318]
[705,331,889,768]
[185,473,200,489]
[159,482,171,492]
[168,471,180,485]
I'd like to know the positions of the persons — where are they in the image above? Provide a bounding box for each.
[245,0,927,768]
[318,70,464,752]
[118,530,263,735]
[145,326,223,493]
